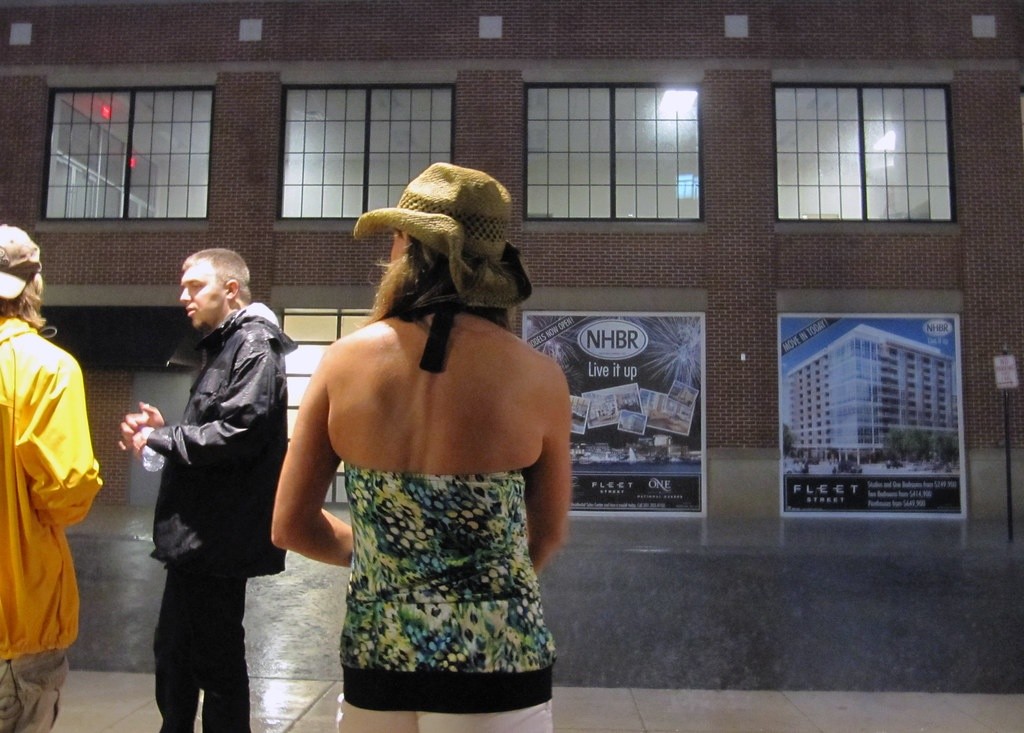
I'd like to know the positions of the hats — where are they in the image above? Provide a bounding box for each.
[354,162,533,308]
[0,223,41,300]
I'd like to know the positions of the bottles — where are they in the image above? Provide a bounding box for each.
[142,445,165,472]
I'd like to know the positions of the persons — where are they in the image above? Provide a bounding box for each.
[1,226,105,733]
[270,163,572,733]
[119,248,297,733]
